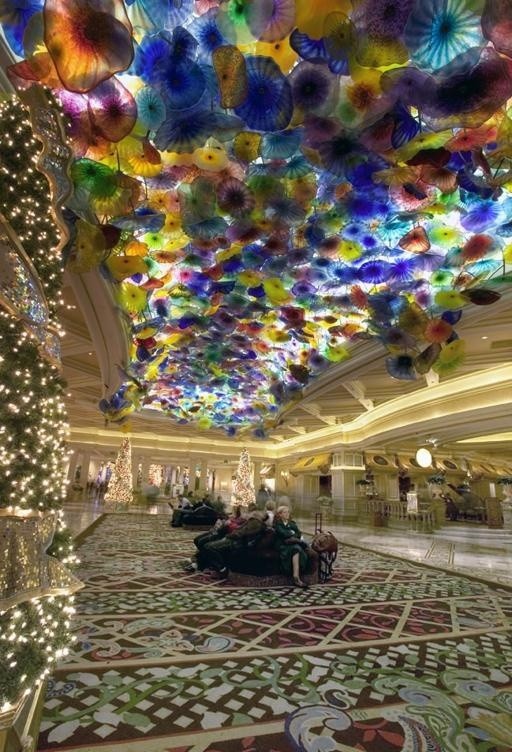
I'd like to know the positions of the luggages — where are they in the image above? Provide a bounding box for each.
[315,512,338,583]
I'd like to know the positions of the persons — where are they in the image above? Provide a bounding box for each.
[167,473,312,588]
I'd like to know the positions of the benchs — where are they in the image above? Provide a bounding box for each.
[178,513,222,533]
[223,546,318,589]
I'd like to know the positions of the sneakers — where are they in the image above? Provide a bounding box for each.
[211,568,230,580]
[292,578,308,588]
[185,563,201,571]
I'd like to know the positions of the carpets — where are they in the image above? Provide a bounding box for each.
[1,512,511,751]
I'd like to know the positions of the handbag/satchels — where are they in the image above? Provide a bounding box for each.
[312,532,338,552]
